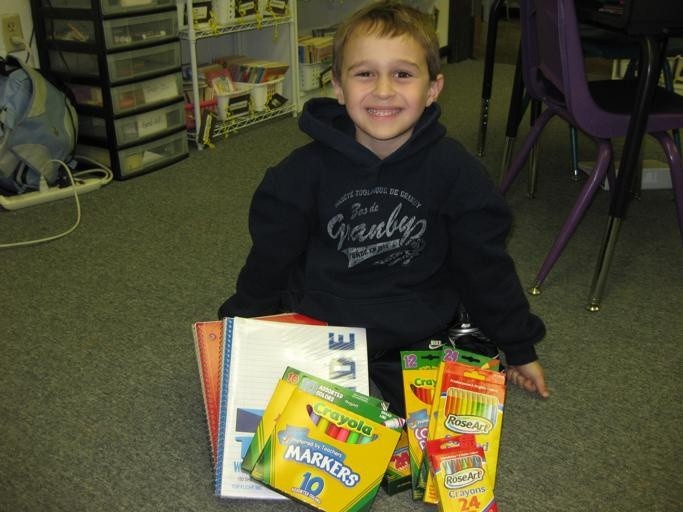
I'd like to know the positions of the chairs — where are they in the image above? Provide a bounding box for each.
[473,0,683,313]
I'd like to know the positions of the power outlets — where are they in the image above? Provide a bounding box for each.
[2,13,28,53]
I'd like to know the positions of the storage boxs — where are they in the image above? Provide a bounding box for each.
[30,1,188,182]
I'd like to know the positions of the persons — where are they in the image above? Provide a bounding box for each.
[218,0,550,423]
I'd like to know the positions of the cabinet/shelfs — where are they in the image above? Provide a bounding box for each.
[175,0,298,141]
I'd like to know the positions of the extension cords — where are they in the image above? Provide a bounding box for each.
[0,178,102,210]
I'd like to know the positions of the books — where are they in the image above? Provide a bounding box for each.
[187,36,334,94]
[191,313,370,501]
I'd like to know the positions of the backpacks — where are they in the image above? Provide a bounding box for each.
[0,55,79,196]
